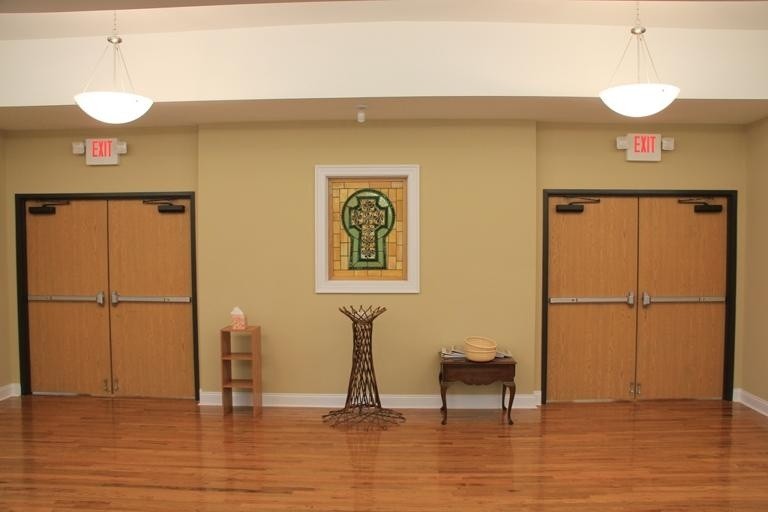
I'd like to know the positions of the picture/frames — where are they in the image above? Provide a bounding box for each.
[314,164,420,294]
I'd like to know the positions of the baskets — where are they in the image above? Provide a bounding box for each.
[463,336,496,362]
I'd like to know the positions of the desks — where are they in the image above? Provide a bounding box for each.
[439,351,517,425]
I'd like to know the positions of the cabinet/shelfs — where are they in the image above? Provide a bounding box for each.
[221,326,263,418]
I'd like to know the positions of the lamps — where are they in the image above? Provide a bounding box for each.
[598,1,681,118]
[72,0,152,124]
